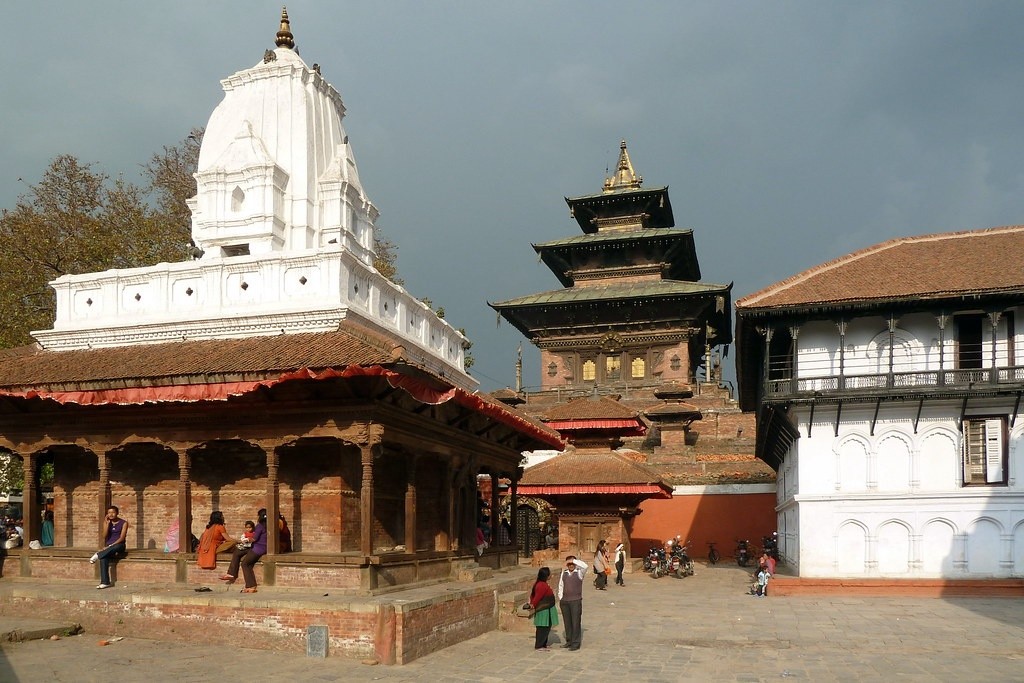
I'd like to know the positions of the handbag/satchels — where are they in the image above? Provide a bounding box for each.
[605,567,612,575]
[28,540,42,549]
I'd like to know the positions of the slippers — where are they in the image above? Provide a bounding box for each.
[194,587,213,592]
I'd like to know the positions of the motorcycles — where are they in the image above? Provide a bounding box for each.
[735,537,750,566]
[762,532,780,561]
[645,535,694,579]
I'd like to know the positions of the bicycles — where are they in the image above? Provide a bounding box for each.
[705,543,720,565]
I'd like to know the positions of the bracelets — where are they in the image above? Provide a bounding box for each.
[107,544,112,547]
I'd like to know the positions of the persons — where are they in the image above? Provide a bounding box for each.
[558,555,589,651]
[89,506,129,589]
[197,511,241,568]
[527,567,559,653]
[41,510,54,546]
[241,521,255,545]
[190,515,201,553]
[614,543,626,587]
[475,518,511,548]
[593,539,610,590]
[278,510,292,554]
[219,508,267,593]
[745,551,776,597]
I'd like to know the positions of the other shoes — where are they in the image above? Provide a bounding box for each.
[90,552,98,563]
[616,581,626,587]
[596,587,606,590]
[568,647,580,651]
[560,643,570,648]
[753,594,765,598]
[241,589,257,593]
[96,584,110,589]
[536,645,551,651]
[219,576,236,580]
